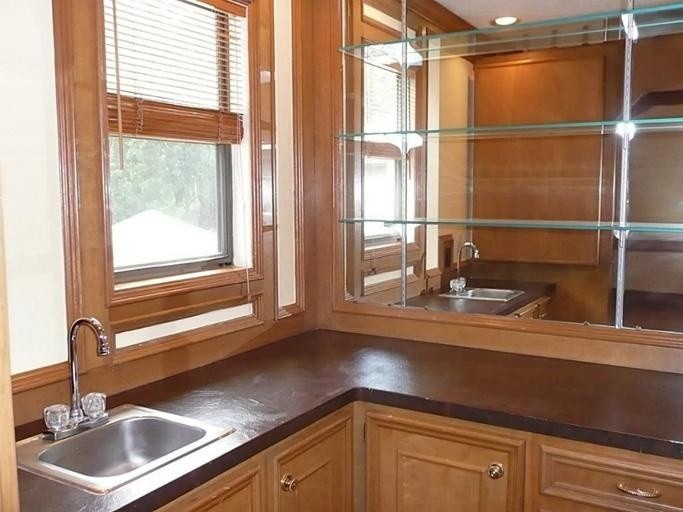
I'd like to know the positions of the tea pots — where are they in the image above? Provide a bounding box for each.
[330,0,681,349]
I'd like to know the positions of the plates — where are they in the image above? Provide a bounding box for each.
[338,2,683,242]
[516,295,552,321]
[524,424,683,509]
[148,452,264,512]
[265,392,358,510]
[359,398,523,509]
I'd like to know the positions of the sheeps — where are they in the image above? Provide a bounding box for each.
[67,316,111,423]
[456,241,480,281]
